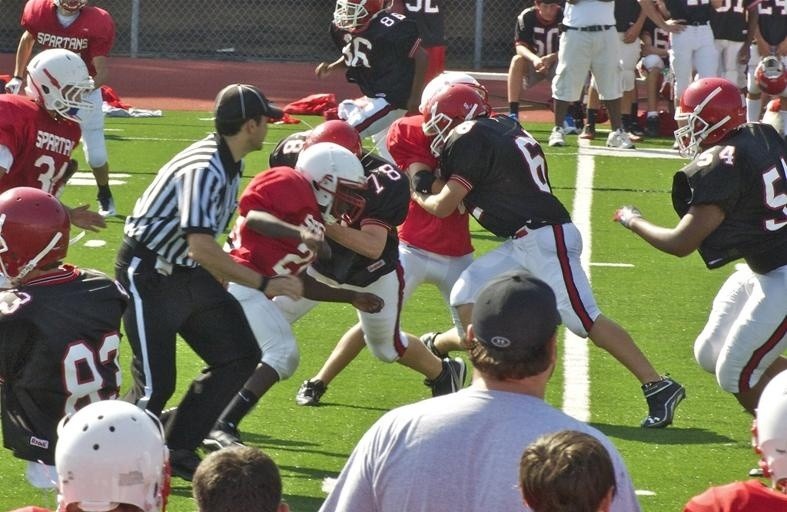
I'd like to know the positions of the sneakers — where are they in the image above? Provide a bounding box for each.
[632,123,644,137]
[419,331,449,360]
[646,117,660,138]
[563,116,576,135]
[581,125,596,139]
[643,374,687,428]
[202,423,246,450]
[295,379,329,407]
[424,357,469,397]
[625,128,640,141]
[606,130,634,150]
[548,126,566,149]
[167,447,202,483]
[96,191,116,220]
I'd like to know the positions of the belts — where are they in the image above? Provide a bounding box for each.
[680,19,708,26]
[122,233,162,267]
[567,23,615,32]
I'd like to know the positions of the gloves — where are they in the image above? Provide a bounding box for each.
[613,204,643,229]
[6,77,24,95]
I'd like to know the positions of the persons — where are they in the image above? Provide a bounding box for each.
[676,370,787,512]
[748,2,787,115]
[2,2,115,217]
[0,44,110,234]
[641,24,671,139]
[748,58,787,139]
[614,79,787,415]
[506,0,577,136]
[327,1,429,113]
[197,144,368,456]
[268,120,467,396]
[707,0,750,87]
[389,0,445,83]
[114,81,285,483]
[294,68,480,409]
[510,430,618,512]
[547,0,637,152]
[581,0,648,140]
[418,70,686,430]
[1,187,129,487]
[3,400,173,512]
[191,444,288,512]
[638,0,719,147]
[315,271,641,512]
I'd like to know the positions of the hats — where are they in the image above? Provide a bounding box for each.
[212,81,285,122]
[473,273,562,347]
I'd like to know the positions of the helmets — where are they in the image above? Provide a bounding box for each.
[418,70,485,114]
[295,142,368,226]
[54,398,168,512]
[754,54,787,94]
[301,119,363,157]
[673,77,746,160]
[24,48,96,124]
[0,187,70,285]
[55,0,89,12]
[750,369,787,494]
[422,84,487,160]
[332,0,384,33]
